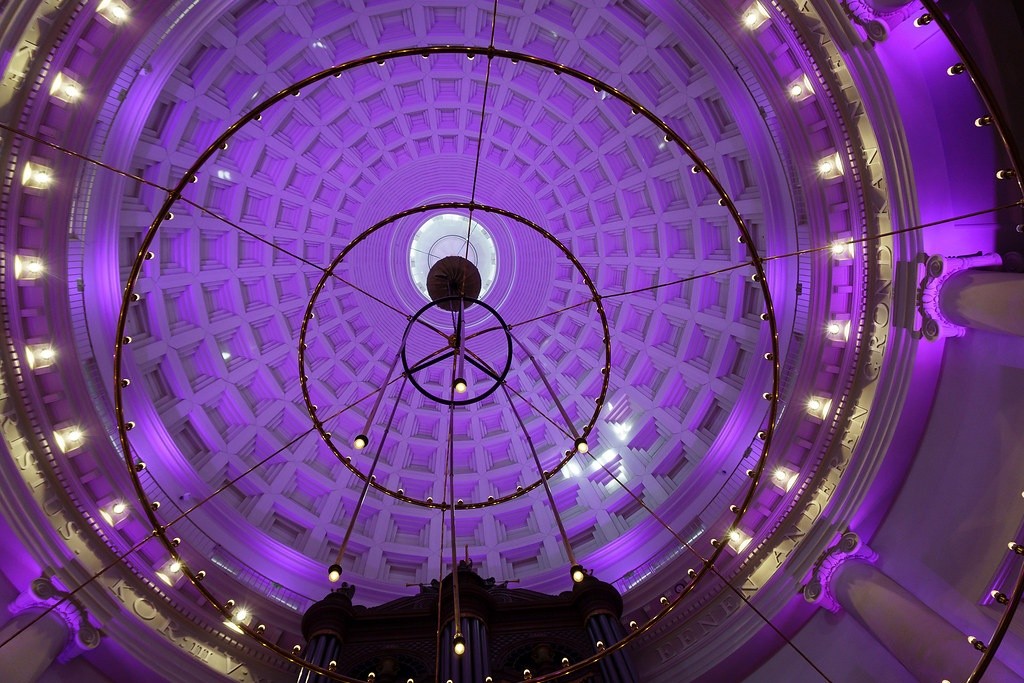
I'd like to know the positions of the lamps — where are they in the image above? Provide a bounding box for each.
[0,0,1024,682]
